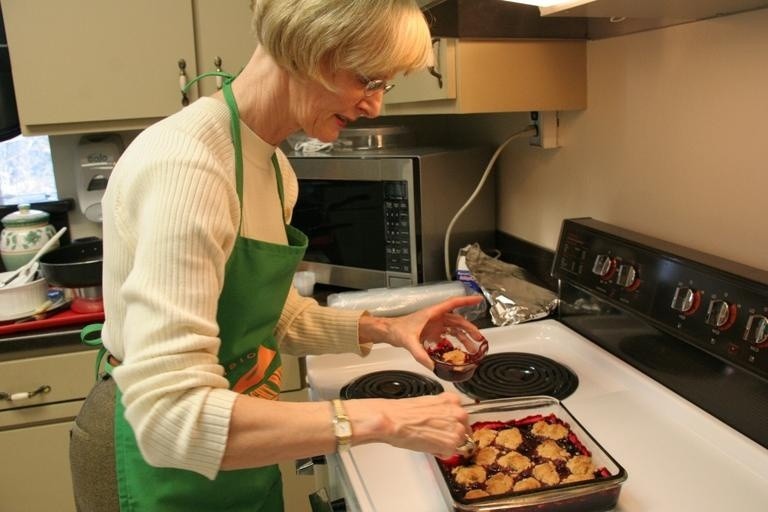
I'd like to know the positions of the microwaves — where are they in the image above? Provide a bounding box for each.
[284,150,498,285]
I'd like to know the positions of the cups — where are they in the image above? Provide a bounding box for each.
[293,269,318,296]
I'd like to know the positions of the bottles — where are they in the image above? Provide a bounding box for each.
[1,203,61,271]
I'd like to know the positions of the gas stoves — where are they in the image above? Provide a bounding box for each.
[300,319,766,511]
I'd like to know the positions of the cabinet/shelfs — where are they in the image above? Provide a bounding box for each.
[0,0,260,137]
[0,350,109,512]
[380,39,587,117]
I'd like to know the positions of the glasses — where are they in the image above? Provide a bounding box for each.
[353,64,395,96]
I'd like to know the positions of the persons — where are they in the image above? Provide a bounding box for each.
[66,0,484,512]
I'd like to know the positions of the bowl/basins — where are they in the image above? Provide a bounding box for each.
[423,324,491,380]
[41,240,103,287]
[0,273,53,316]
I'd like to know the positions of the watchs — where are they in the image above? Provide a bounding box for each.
[330,398,355,453]
